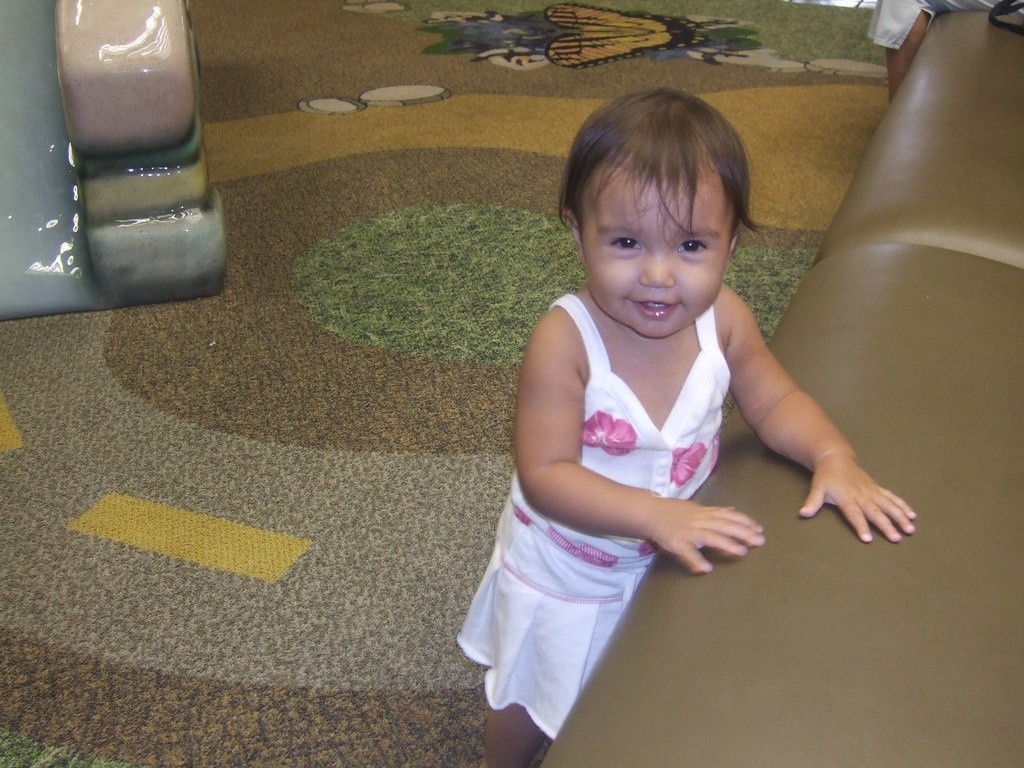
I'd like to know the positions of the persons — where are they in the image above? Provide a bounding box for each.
[455,86,917,768]
[867,0,1024,103]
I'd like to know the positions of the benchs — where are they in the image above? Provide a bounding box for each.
[541,11,1024,768]
